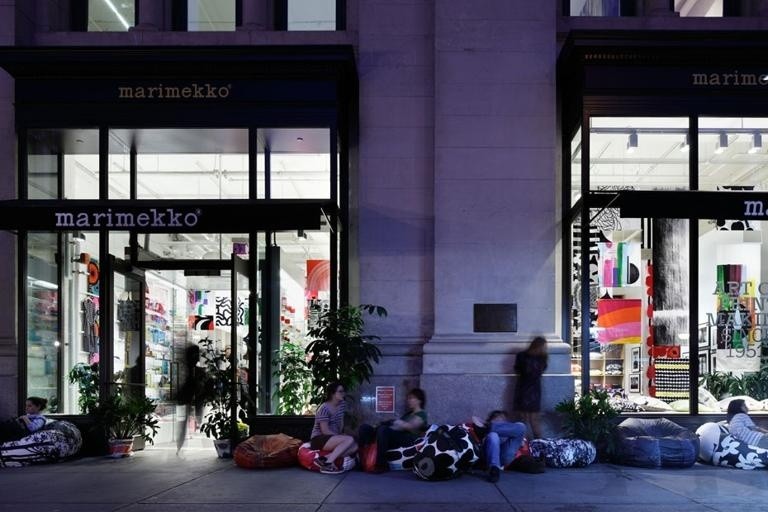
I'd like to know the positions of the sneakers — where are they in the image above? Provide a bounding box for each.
[313,455,345,475]
[472,422,491,441]
[486,466,501,484]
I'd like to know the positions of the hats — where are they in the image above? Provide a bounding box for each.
[485,410,508,424]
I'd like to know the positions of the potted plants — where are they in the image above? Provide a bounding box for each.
[67,361,160,458]
[198,336,254,459]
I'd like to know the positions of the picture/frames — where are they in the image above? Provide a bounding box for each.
[628,347,640,392]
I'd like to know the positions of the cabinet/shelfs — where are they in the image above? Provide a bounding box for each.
[572,339,625,396]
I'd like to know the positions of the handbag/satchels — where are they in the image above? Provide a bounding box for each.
[0,417,47,444]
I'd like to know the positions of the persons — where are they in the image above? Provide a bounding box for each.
[170,339,214,448]
[0,397,48,445]
[513,336,548,439]
[472,411,526,483]
[310,382,359,474]
[358,388,427,475]
[727,399,768,451]
